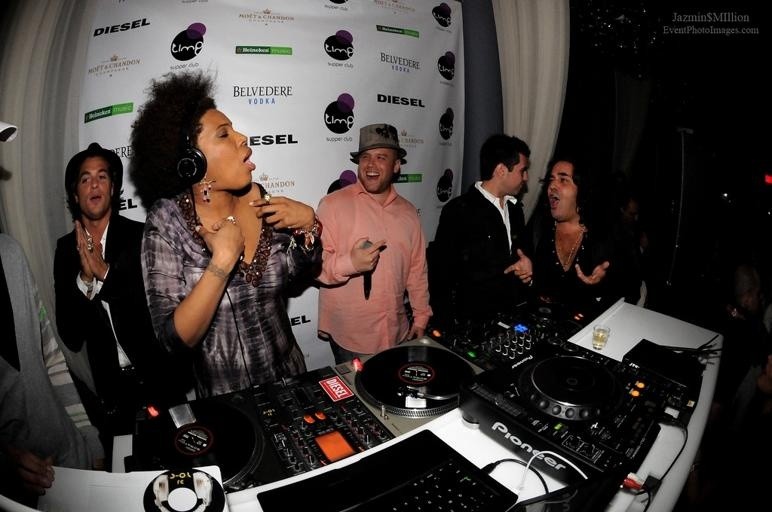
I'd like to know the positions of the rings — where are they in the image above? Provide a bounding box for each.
[86,233,93,251]
[75,246,80,252]
[264,194,271,205]
[226,215,235,222]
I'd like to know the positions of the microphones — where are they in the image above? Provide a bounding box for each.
[360,241,374,300]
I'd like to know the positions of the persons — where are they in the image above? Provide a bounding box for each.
[54,143,179,468]
[604,193,772,509]
[503,149,630,321]
[425,132,531,330]
[0,123,102,507]
[317,123,434,364]
[130,73,326,399]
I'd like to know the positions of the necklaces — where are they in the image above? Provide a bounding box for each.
[552,224,585,271]
[170,182,271,287]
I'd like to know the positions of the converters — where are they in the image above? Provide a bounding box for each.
[642,475,662,494]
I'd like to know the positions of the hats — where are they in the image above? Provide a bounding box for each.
[348,122,409,162]
[65,142,124,208]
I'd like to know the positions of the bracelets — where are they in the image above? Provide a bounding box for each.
[294,212,322,253]
[206,260,229,281]
[83,280,94,292]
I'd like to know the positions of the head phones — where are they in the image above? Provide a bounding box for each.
[174,131,208,185]
